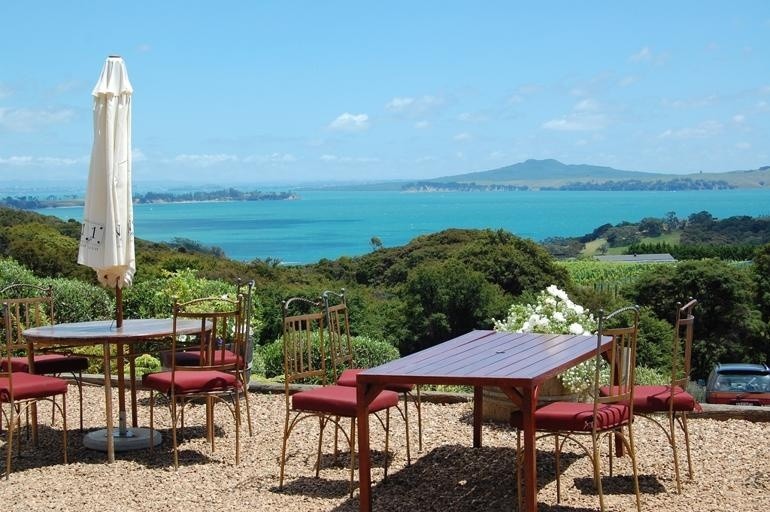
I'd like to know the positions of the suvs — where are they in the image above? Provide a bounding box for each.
[700,358,770,408]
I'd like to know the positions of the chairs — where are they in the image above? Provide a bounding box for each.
[597,292,700,495]
[506,303,644,512]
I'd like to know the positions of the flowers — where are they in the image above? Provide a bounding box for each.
[482,283,618,397]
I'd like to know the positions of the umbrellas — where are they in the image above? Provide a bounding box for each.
[75,53,165,435]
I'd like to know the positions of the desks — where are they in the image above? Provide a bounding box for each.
[348,324,628,512]
